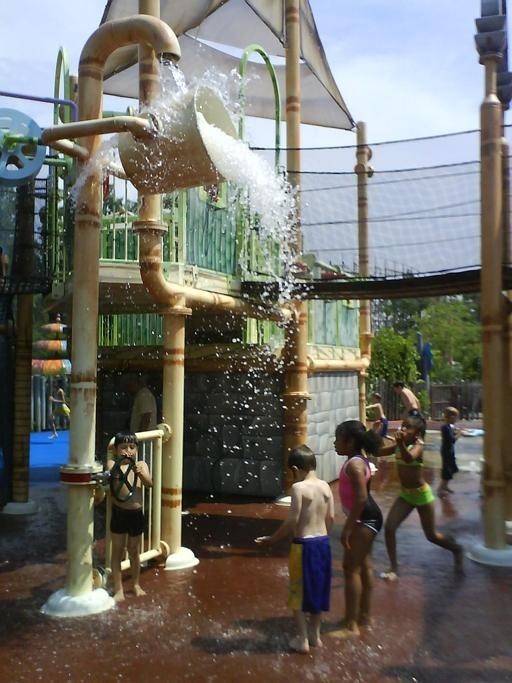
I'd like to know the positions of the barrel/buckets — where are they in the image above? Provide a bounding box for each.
[116,88,239,195]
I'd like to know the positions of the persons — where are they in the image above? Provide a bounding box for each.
[326,420,386,638]
[254,444,335,655]
[365,392,394,442]
[127,373,158,465]
[371,414,463,577]
[440,407,459,493]
[392,382,421,417]
[106,431,152,602]
[48,376,71,440]
